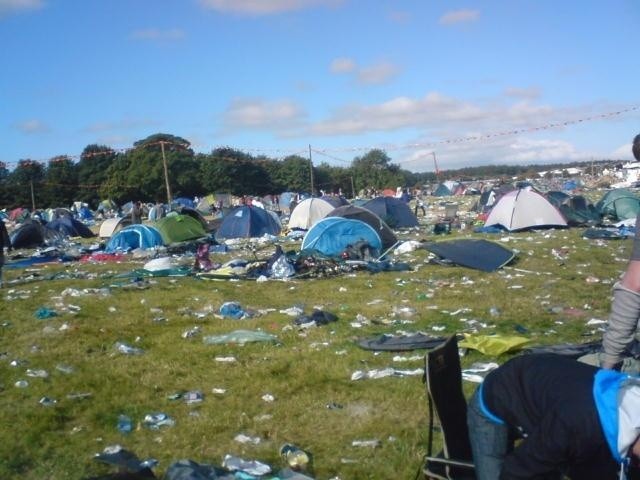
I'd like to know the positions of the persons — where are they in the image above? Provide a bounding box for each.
[597,133,640,371]
[415,192,426,217]
[461,350,640,479]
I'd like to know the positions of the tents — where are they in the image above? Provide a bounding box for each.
[472,187,639,233]
[357,167,639,213]
[1,192,418,262]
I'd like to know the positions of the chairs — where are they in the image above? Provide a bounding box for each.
[419,332,484,480]
